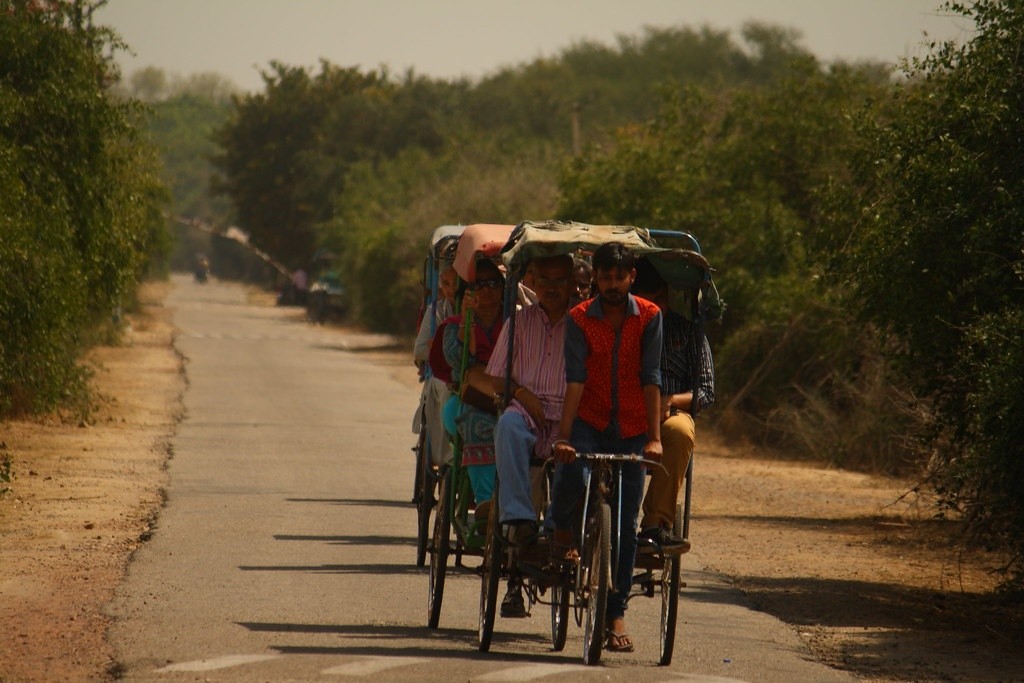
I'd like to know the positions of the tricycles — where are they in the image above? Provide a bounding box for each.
[411,225,651,571]
[473,221,714,668]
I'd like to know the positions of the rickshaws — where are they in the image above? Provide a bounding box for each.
[424,224,699,651]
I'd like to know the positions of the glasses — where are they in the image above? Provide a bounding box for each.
[475,277,503,290]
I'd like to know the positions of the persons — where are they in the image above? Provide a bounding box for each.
[541,240,665,654]
[410,262,469,498]
[570,257,595,308]
[626,275,716,555]
[517,256,544,312]
[428,259,516,539]
[483,255,576,549]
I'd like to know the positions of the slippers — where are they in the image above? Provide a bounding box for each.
[474,500,690,651]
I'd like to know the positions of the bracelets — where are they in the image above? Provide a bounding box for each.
[550,439,571,452]
[514,387,527,400]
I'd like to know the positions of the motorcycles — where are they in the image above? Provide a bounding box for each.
[195,253,209,283]
